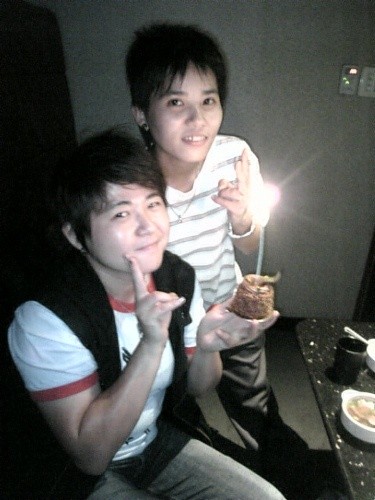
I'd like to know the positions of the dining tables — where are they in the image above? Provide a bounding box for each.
[296,317,375,500]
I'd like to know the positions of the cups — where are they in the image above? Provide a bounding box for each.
[335,337,367,385]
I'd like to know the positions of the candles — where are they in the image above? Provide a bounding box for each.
[255,228,265,281]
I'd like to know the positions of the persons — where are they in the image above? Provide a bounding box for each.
[124,22,311,492]
[7,128,288,500]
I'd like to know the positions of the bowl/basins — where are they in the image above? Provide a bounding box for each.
[341,389,375,442]
[366,339,375,372]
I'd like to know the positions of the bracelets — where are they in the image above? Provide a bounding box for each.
[227,216,256,239]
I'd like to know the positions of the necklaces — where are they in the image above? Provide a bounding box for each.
[165,189,196,224]
